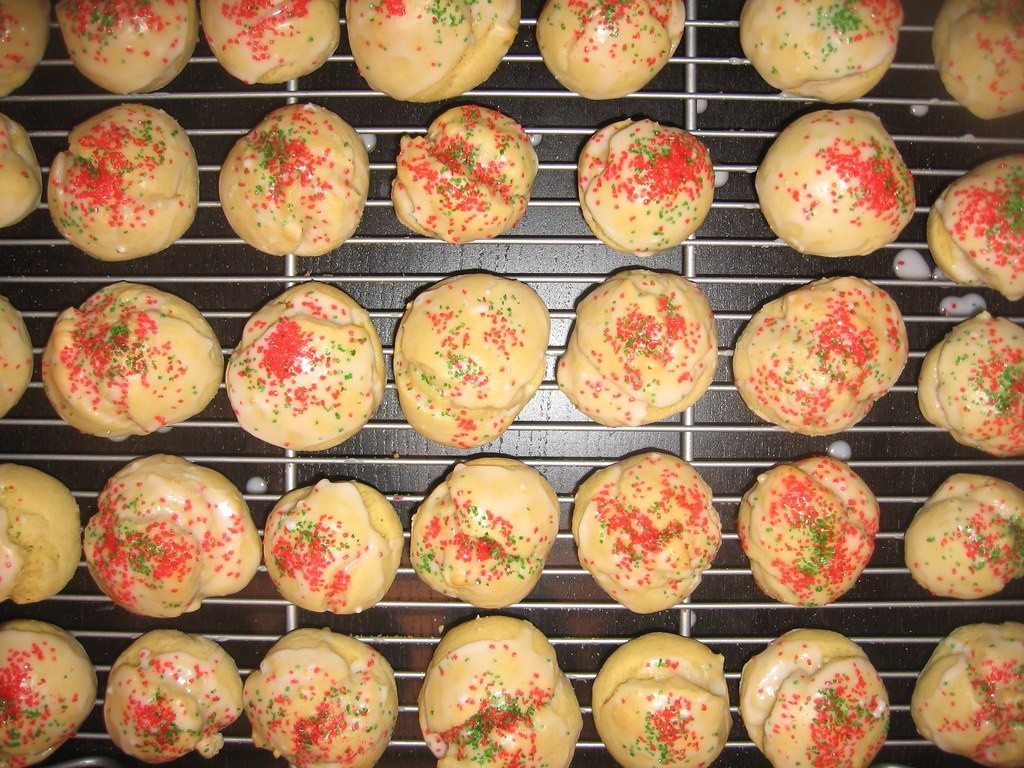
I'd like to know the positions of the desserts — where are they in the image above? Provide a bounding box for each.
[0,0,1024,768]
